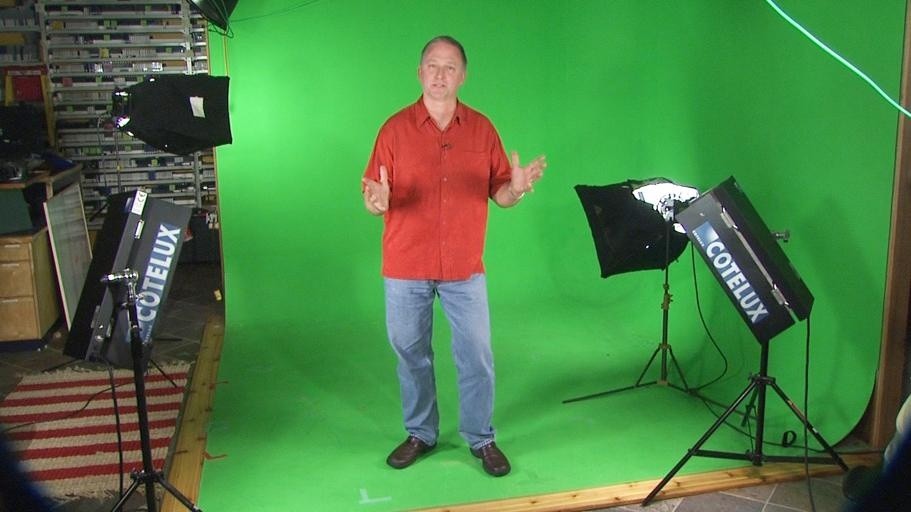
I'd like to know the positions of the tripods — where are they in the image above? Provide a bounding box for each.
[640,342,850,507]
[635,222,689,393]
[110,303,201,512]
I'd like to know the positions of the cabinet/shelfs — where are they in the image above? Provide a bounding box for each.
[0,0,220,353]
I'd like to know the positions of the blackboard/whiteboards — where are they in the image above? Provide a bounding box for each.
[43,182,93,332]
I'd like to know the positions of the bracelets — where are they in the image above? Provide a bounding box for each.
[509,181,526,200]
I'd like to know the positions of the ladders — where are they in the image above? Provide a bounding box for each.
[5,66,60,153]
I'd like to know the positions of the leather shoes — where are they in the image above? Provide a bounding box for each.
[469,440,510,477]
[386,434,437,469]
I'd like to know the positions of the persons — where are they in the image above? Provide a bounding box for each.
[359,32,548,477]
[841,392,911,499]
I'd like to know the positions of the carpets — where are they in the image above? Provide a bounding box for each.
[1,360,195,504]
